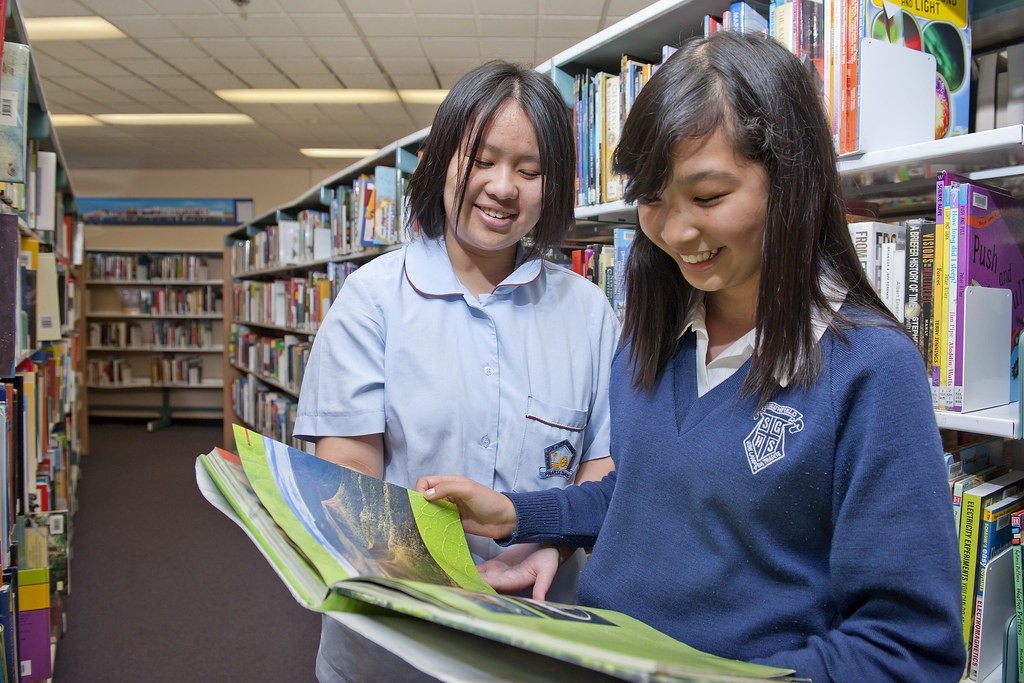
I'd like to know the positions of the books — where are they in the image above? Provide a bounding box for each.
[196,424,795,683]
[229,0,1024,683]
[0,0,216,683]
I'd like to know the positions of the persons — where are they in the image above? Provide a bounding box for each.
[294,58,622,683]
[420,31,968,683]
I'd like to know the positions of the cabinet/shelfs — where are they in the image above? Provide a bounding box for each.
[0,0,1024,682]
